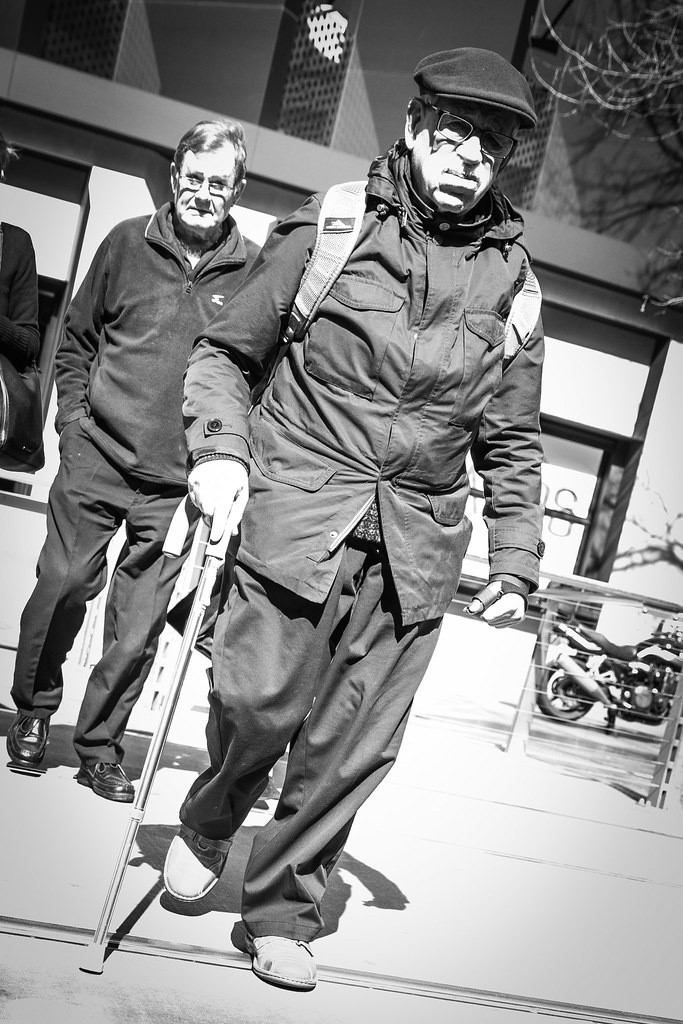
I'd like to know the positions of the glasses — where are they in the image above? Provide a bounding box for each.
[177,169,235,195]
[425,103,519,159]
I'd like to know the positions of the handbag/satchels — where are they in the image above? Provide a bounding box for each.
[0,353,46,474]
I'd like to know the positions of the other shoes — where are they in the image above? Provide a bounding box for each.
[242,932,318,990]
[163,822,242,903]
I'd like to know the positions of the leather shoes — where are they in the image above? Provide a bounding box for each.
[6,710,49,768]
[76,761,135,803]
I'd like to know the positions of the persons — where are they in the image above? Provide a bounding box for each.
[6,119,266,806]
[0,221,41,369]
[166,46,543,992]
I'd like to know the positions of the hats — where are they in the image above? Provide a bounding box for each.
[412,47,538,129]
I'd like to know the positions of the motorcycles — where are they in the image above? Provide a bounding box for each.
[537,612,683,734]
